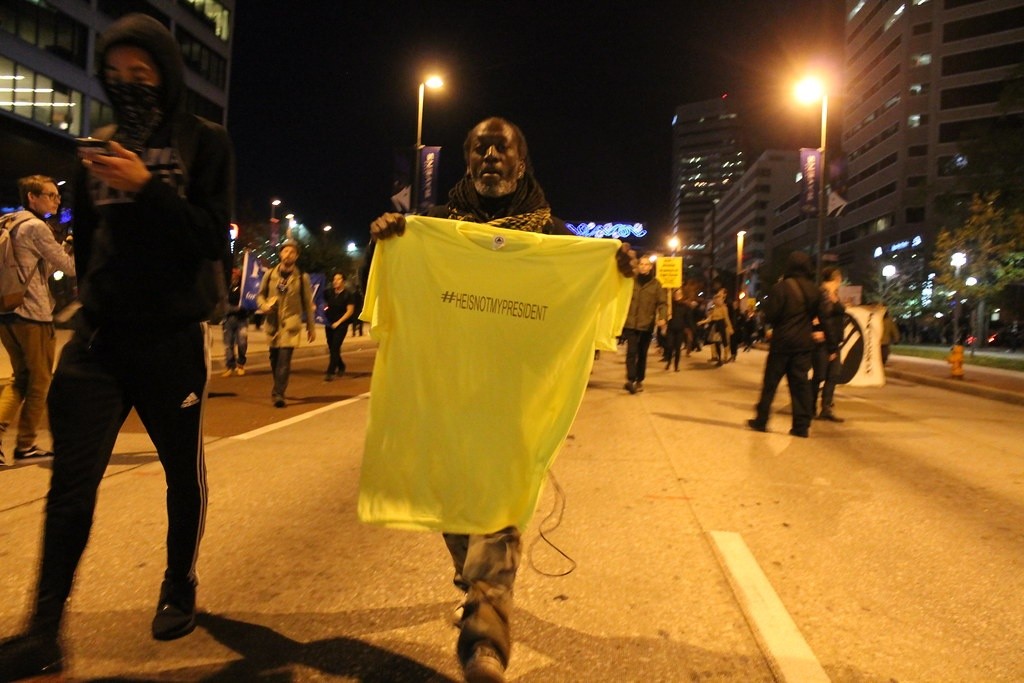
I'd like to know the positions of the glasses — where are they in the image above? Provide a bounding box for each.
[38,192,62,201]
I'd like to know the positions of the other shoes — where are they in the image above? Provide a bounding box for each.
[819,407,844,423]
[337,365,345,377]
[221,366,234,378]
[273,391,284,407]
[152,579,197,639]
[749,419,765,432]
[325,373,334,382]
[0,634,63,681]
[624,381,643,393]
[236,363,244,376]
[790,429,807,437]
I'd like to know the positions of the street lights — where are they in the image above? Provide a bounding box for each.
[412,74,446,216]
[950,252,967,344]
[271,198,282,246]
[735,228,747,319]
[791,64,830,288]
[708,197,721,299]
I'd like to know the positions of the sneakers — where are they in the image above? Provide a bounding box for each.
[454,591,468,627]
[14,445,54,464]
[463,646,506,682]
[0,449,8,466]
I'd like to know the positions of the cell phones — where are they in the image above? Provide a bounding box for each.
[75,137,112,163]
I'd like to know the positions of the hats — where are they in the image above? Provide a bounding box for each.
[277,239,301,261]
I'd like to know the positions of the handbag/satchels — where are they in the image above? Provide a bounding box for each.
[810,307,825,343]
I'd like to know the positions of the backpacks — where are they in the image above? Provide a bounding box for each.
[0,212,44,312]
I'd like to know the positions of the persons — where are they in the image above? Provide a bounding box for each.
[255,239,316,408]
[616,255,766,395]
[0,173,76,466]
[880,313,900,366]
[322,274,355,381]
[212,268,249,376]
[356,116,659,683]
[351,285,363,337]
[0,14,232,683]
[747,250,847,441]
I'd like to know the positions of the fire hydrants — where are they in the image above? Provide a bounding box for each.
[944,342,965,381]
